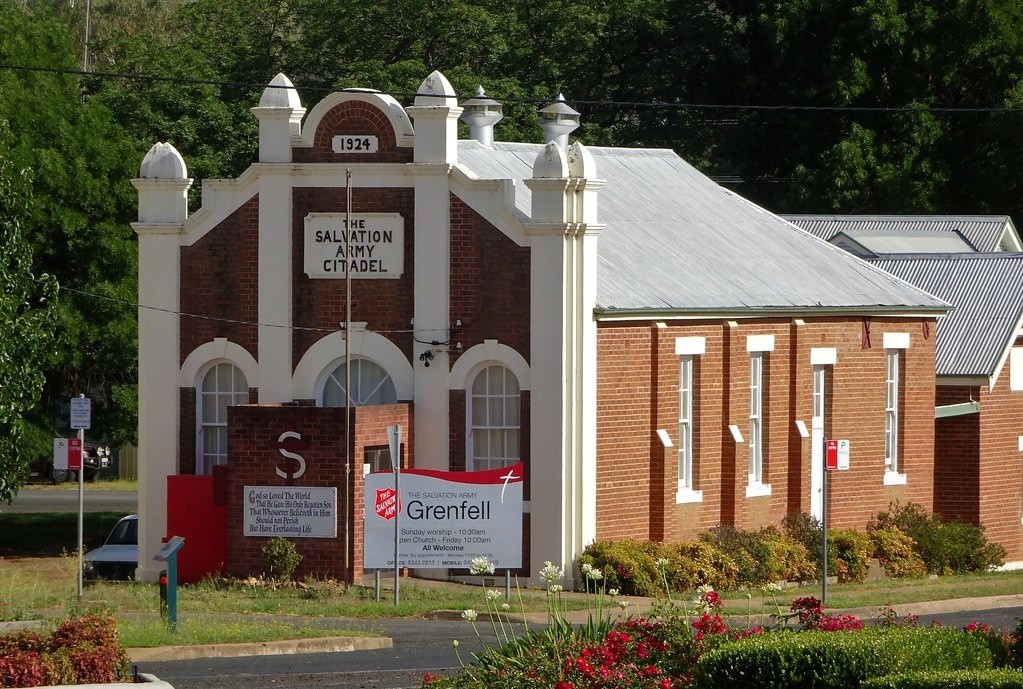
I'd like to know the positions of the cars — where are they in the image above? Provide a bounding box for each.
[82,514,139,584]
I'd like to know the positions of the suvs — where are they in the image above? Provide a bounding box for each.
[29,429,113,484]
